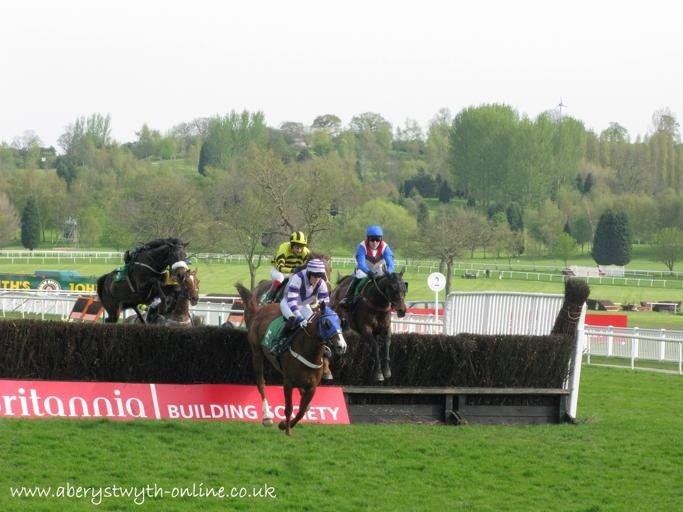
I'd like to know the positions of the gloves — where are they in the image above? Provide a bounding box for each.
[367,270,378,280]
[299,319,307,328]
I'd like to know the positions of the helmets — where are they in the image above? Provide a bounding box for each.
[290,231,307,244]
[307,258,325,273]
[366,225,384,236]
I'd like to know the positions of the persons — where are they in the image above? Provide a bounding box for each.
[338,225,395,309]
[258,231,312,307]
[269,258,330,358]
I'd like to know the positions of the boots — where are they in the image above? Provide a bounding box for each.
[339,277,361,307]
[266,292,274,304]
[270,337,287,356]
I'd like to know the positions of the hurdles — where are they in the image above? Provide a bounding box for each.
[0,278,591,425]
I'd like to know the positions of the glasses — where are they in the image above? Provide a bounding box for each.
[312,273,322,277]
[370,238,380,242]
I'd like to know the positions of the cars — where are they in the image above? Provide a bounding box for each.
[403,301,444,317]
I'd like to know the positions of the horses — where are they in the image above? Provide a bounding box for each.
[93,236,201,326]
[230,251,411,437]
